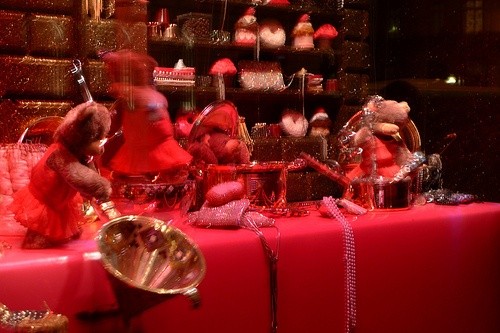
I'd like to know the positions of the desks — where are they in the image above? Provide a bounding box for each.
[0,195,500,332]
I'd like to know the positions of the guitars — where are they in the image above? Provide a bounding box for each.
[64,58,93,102]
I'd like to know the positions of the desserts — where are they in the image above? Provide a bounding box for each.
[290,13,315,49]
[232,5,259,47]
[309,106,332,137]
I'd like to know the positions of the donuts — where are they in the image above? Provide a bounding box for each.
[259,18,286,48]
[279,108,308,138]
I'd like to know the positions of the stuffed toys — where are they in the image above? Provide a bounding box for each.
[14,102,113,249]
[354,95,413,179]
